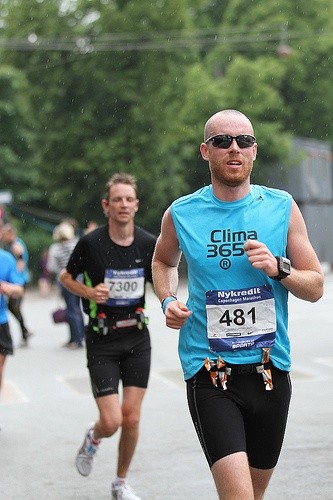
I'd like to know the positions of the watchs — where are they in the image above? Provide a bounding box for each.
[269,256,291,281]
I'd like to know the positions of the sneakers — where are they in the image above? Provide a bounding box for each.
[112,482,141,500]
[75,428,100,476]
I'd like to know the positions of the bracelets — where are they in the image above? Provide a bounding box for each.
[160,293,177,314]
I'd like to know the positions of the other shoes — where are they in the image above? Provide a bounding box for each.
[65,341,79,349]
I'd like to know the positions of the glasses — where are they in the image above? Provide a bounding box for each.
[205,134,255,149]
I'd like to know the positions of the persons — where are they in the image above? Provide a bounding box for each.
[57,172,157,500]
[44,217,99,349]
[0,218,32,392]
[152,110,324,500]
